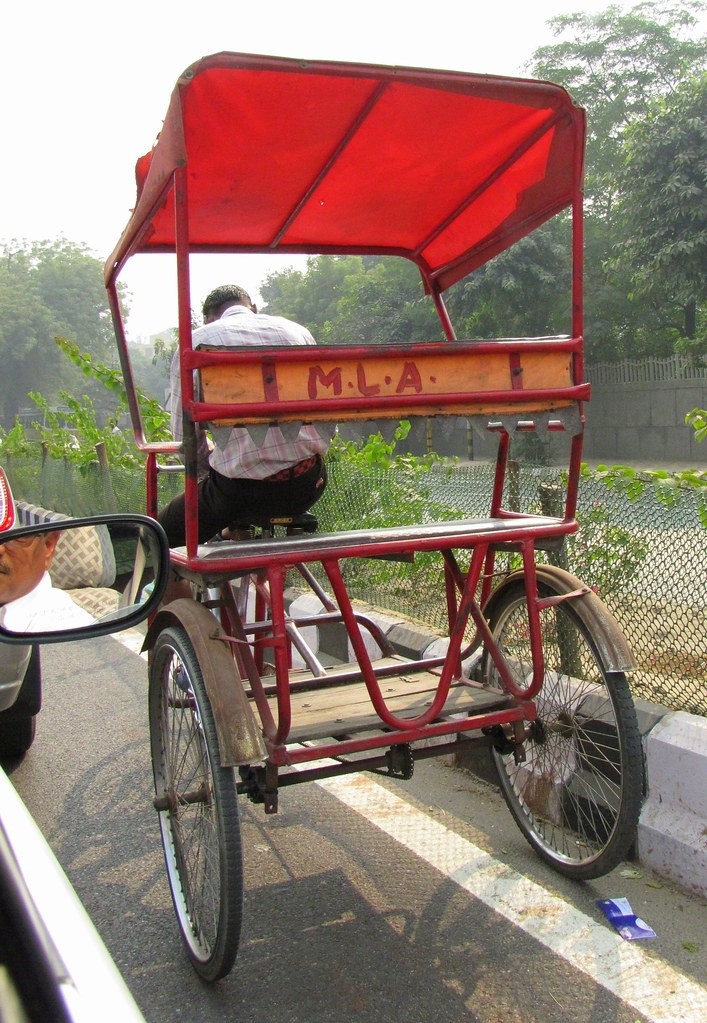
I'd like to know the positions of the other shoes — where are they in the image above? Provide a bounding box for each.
[173,664,192,694]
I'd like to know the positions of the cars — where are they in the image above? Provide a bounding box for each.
[0,465,43,756]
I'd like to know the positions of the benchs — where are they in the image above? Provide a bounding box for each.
[160,331,575,588]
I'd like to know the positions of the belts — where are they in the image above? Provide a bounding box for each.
[265,453,318,482]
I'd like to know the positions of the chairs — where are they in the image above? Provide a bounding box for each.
[50,523,126,621]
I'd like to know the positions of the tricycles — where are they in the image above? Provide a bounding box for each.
[105,52,649,984]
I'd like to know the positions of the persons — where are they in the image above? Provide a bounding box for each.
[0,530,97,633]
[155,285,329,691]
